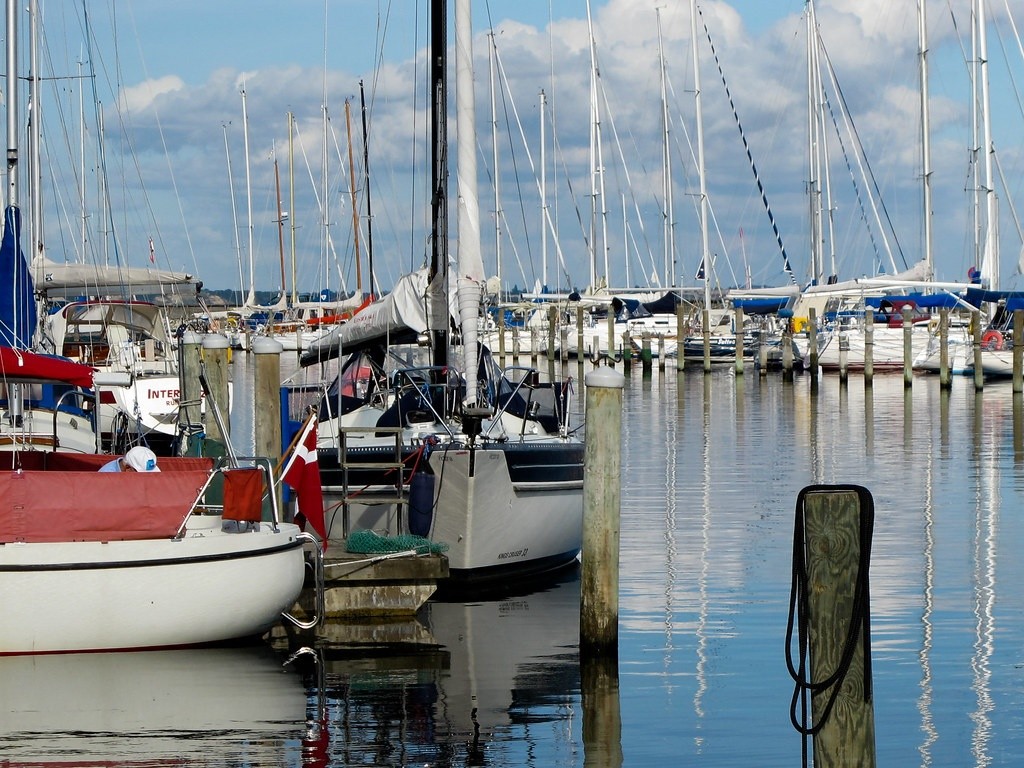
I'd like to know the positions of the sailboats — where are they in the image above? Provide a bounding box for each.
[1,1,1024,659]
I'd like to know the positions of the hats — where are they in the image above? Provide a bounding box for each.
[122,445,162,473]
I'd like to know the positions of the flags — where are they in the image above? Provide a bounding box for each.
[280,414,328,554]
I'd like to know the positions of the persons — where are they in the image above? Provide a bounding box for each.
[98,446,162,472]
